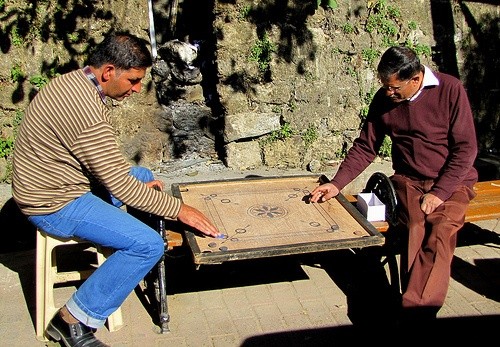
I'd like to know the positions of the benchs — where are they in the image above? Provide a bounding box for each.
[153,172,500,336]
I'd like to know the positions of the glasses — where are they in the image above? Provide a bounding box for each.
[378,75,413,92]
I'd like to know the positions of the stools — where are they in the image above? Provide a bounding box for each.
[35,229,126,343]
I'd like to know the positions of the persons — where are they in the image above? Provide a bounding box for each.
[10,29,222,346]
[306,46,478,347]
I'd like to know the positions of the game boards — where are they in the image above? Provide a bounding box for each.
[171,174,385,263]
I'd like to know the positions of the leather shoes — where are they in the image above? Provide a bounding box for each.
[44,309,109,347]
